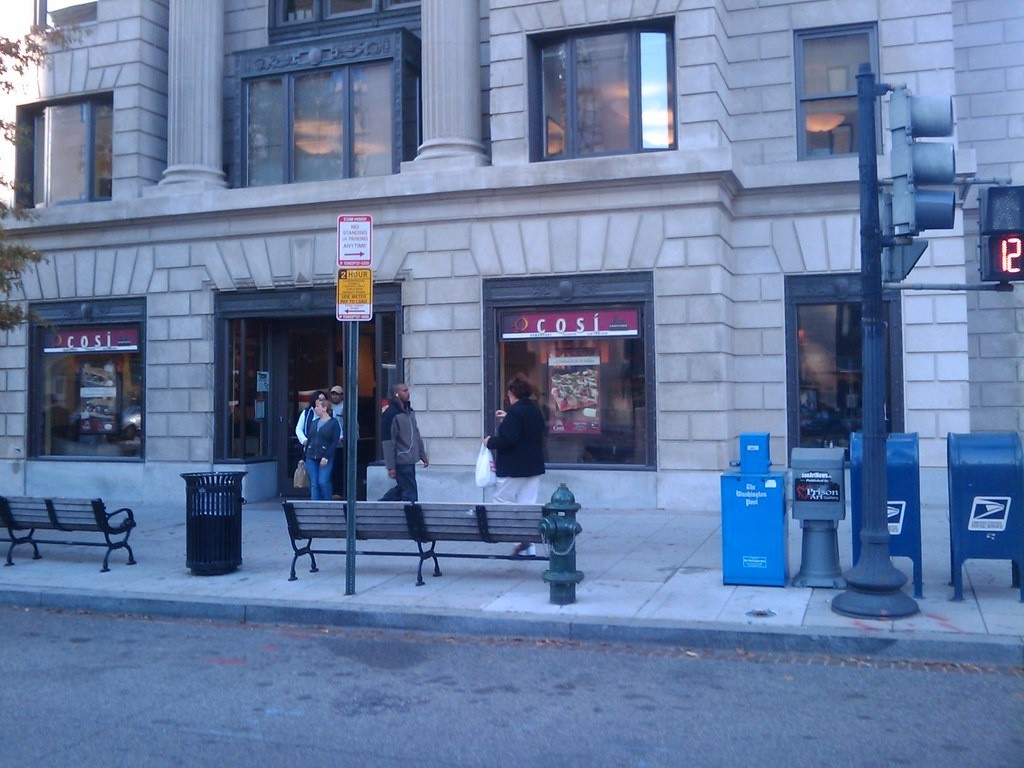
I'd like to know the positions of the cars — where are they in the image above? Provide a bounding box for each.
[122,405,141,439]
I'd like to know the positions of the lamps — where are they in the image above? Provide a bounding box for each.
[807,112,845,134]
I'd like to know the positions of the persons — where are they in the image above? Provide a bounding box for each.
[296,384,360,500]
[86,405,111,416]
[118,422,140,457]
[375,382,428,502]
[484,375,547,556]
[43,394,69,436]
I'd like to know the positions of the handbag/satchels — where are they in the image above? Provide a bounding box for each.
[292,461,310,489]
[474,436,498,488]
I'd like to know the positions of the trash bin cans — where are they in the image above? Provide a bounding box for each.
[849,432,924,601]
[181,471,249,577]
[719,433,790,588]
[946,431,1024,606]
[790,448,847,590]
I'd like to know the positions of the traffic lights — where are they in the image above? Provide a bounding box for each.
[881,241,929,282]
[890,88,956,238]
[977,185,1023,283]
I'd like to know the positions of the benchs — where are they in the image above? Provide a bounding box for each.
[0,495,137,572]
[280,491,551,586]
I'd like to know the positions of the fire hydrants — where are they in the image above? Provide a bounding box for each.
[535,483,586,602]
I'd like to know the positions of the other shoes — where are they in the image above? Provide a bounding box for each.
[508,542,537,556]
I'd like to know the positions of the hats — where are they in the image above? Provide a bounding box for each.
[330,385,343,394]
